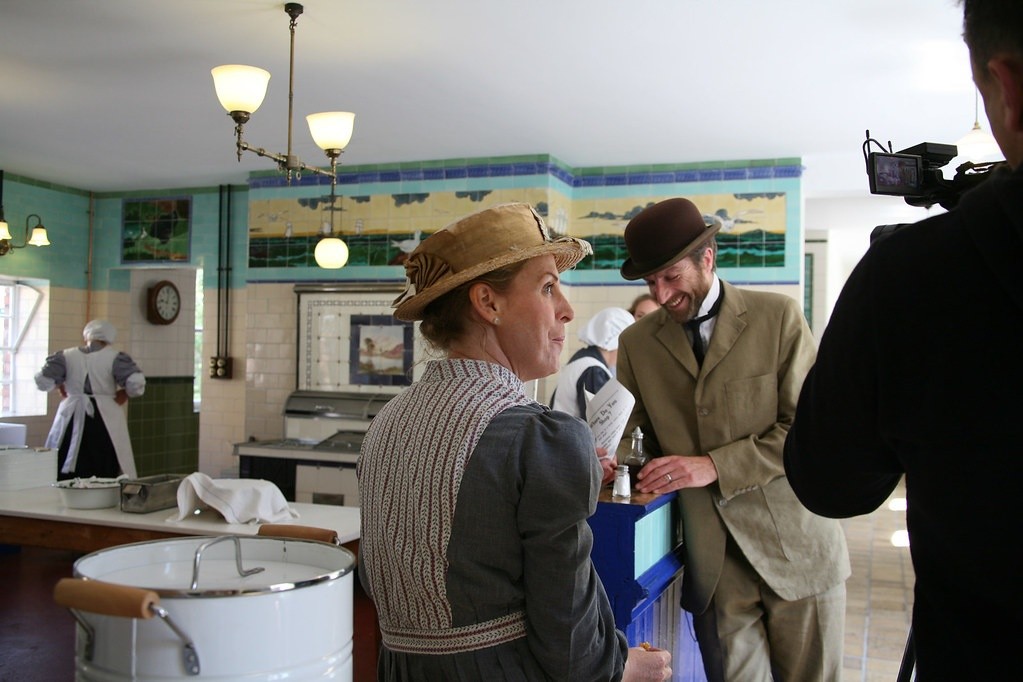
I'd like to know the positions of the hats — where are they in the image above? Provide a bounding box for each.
[577,306,637,352]
[618,198,723,281]
[390,203,594,323]
[82,319,117,345]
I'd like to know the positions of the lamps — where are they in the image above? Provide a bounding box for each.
[210,2,356,186]
[315,181,351,273]
[0,169,50,257]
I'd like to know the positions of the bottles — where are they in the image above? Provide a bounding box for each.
[612,463,631,499]
[625,426,649,492]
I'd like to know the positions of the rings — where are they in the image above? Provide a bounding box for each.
[666,473,672,481]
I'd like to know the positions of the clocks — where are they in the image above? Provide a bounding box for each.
[145,276,184,326]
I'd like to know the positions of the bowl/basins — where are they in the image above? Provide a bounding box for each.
[51,478,121,510]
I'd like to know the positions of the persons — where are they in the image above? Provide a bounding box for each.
[549,307,635,422]
[356,203,673,682]
[780,0,1023,682]
[34,318,146,480]
[615,196,852,682]
[628,293,660,321]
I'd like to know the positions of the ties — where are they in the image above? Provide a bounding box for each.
[683,278,724,370]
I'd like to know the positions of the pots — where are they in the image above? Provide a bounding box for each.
[52,524,357,682]
